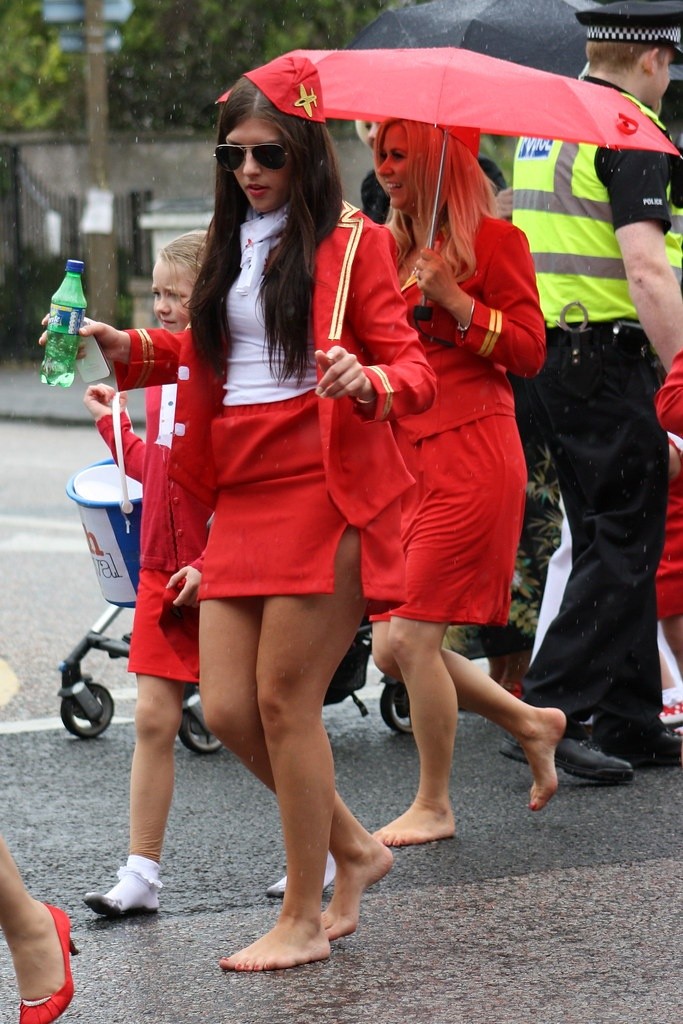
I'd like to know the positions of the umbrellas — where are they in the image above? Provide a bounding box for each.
[344,0,605,79]
[215,46,683,160]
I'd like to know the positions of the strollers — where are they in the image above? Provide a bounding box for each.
[60,456,415,755]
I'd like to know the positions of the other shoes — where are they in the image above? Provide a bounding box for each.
[658,701,683,729]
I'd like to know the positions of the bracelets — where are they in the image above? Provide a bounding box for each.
[457,298,475,339]
[357,397,375,403]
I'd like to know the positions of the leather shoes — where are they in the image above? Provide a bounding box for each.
[500,722,635,783]
[613,728,682,769]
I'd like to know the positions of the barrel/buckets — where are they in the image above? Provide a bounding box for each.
[65,392,143,608]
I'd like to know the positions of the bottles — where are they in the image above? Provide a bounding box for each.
[39,260,87,388]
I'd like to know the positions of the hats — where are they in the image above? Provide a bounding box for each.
[440,122,482,158]
[575,0,683,56]
[244,54,327,123]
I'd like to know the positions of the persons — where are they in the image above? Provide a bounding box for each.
[354,120,512,226]
[442,0,683,783]
[83,230,337,917]
[39,57,438,971]
[0,833,80,1024]
[372,117,567,847]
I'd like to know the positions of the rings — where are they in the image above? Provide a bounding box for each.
[415,269,419,278]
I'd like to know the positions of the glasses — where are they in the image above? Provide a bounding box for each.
[213,140,290,172]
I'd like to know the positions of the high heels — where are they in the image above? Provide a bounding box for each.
[18,904,80,1024]
